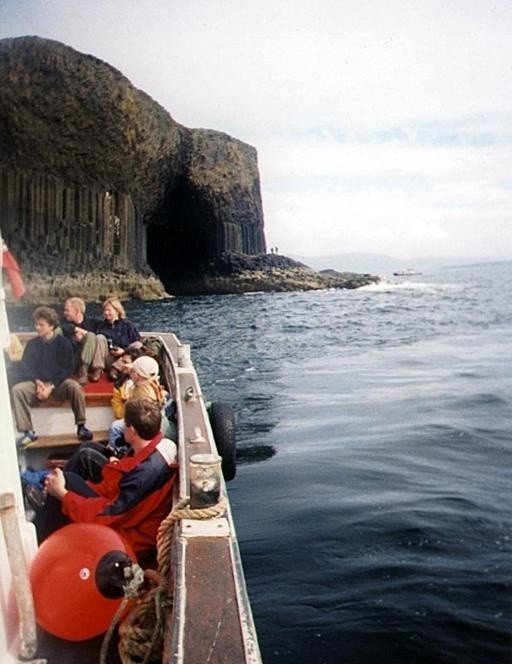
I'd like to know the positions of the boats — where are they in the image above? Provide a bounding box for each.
[392,267,423,276]
[0,231,267,664]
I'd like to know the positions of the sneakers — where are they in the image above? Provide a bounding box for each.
[77,367,88,384]
[108,366,121,380]
[16,432,39,450]
[77,428,93,441]
[91,368,105,381]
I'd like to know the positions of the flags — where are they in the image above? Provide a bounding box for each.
[1,234,27,298]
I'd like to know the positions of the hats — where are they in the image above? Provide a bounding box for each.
[122,356,160,380]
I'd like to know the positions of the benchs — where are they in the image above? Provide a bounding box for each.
[5,339,175,433]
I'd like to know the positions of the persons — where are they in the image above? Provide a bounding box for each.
[61,296,95,379]
[108,332,177,450]
[12,304,92,443]
[31,396,179,556]
[90,296,137,383]
